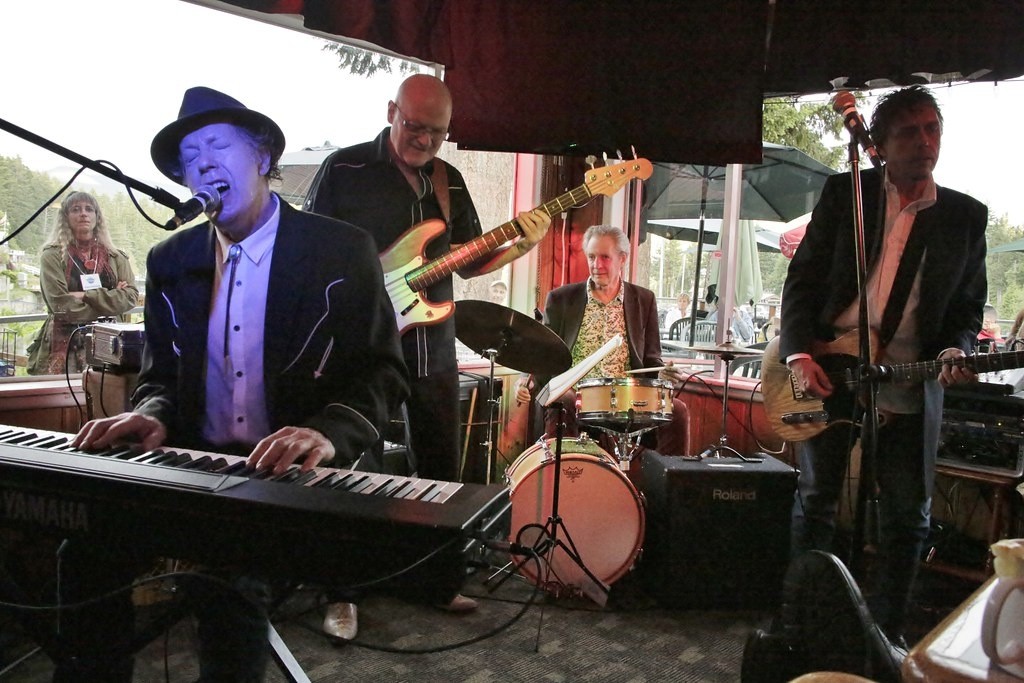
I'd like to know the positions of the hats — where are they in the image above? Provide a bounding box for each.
[492,280,507,289]
[151,86,286,188]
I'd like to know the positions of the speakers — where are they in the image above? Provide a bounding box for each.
[639,449,801,611]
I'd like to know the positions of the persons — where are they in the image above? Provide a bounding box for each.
[978,301,1024,352]
[704,296,754,344]
[300,74,551,641]
[489,280,508,304]
[25,191,139,374]
[516,225,690,456]
[697,300,709,317]
[662,292,691,350]
[46,87,412,683]
[775,88,990,683]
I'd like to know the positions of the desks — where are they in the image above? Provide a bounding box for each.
[898,575,1023,683]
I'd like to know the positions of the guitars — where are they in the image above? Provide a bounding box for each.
[760,326,1024,443]
[379,145,654,338]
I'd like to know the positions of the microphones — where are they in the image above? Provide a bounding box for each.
[831,91,884,178]
[166,184,221,231]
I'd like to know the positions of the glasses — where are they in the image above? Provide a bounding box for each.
[394,103,451,141]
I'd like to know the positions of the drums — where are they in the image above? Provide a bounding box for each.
[574,376,675,426]
[502,433,646,599]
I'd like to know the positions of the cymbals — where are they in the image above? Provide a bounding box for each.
[454,299,573,375]
[683,343,764,358]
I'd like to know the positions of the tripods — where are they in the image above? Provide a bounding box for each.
[488,333,625,613]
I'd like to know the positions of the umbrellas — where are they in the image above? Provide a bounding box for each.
[987,238,1024,253]
[641,140,842,347]
[269,139,340,206]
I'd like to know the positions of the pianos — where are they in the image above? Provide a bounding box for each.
[0,424,513,683]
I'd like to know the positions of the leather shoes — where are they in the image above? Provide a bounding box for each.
[740,628,798,683]
[782,551,909,683]
[436,593,478,610]
[324,603,358,640]
